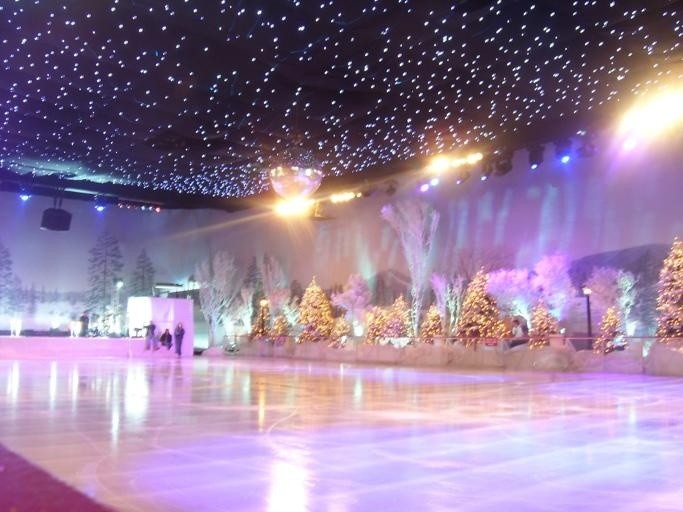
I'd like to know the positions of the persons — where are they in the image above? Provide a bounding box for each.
[77,312,89,336]
[158,328,173,351]
[145,320,160,350]
[511,319,524,338]
[173,321,185,357]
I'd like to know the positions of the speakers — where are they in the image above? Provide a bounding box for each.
[40,208,72,232]
